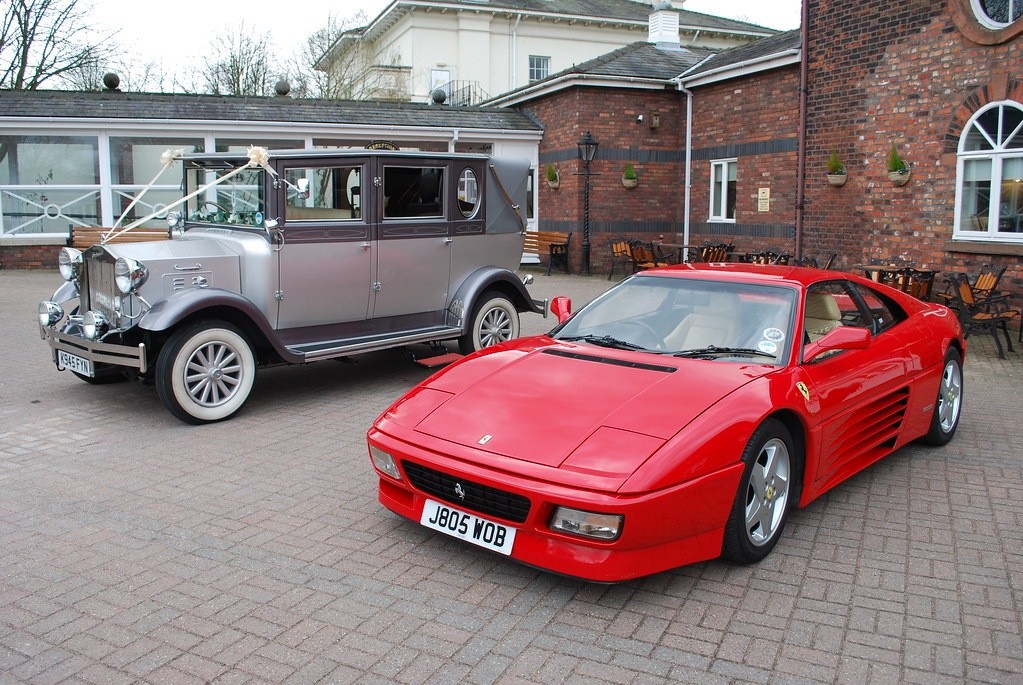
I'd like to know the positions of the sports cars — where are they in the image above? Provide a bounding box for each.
[364,259,968,584]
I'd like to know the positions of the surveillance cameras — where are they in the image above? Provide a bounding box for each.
[637,115,644,123]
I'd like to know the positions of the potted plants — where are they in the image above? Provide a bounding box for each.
[825,148,848,186]
[623,162,637,189]
[544,162,559,190]
[887,141,912,186]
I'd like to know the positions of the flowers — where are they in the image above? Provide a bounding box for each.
[191,206,256,225]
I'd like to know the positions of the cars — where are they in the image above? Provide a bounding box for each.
[37,147,551,427]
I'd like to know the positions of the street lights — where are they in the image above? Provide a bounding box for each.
[572,129,602,276]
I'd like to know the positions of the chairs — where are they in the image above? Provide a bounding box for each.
[606,236,934,308]
[949,272,1019,360]
[935,263,1014,316]
[753,293,844,363]
[647,292,746,352]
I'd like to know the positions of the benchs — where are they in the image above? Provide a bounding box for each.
[65,225,174,250]
[523,229,573,278]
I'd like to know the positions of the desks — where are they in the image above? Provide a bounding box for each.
[725,250,791,262]
[854,263,933,284]
[656,243,708,265]
